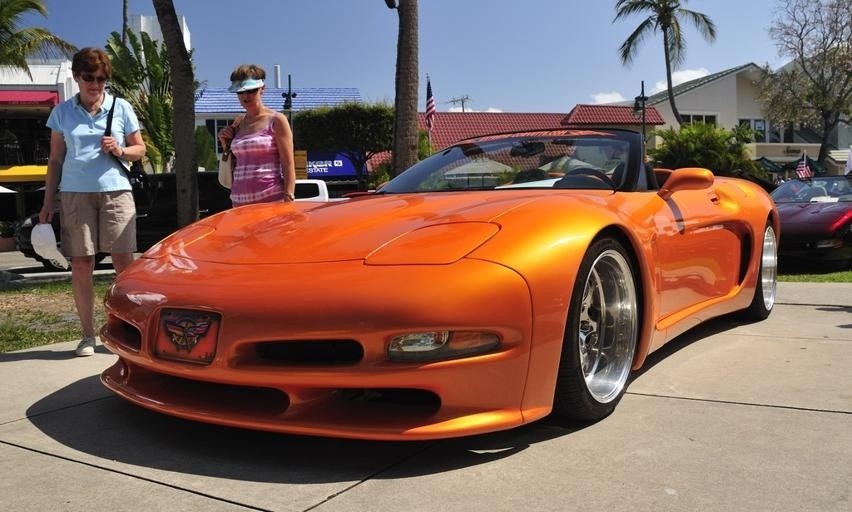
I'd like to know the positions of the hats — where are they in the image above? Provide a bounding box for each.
[227,78,265,93]
[29,222,69,270]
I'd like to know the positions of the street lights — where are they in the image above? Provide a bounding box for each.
[281,74,297,133]
[632,80,649,140]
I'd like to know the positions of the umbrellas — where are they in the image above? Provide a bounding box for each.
[752,156,784,175]
[784,156,828,172]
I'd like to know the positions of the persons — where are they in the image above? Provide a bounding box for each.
[774,175,785,186]
[37,48,148,358]
[217,64,295,208]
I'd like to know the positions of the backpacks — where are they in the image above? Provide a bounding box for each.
[125,161,155,213]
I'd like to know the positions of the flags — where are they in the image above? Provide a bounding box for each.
[795,152,813,180]
[426,80,435,132]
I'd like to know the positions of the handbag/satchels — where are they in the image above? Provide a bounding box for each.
[217,125,238,190]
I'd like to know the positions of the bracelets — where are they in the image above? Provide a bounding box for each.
[119,147,125,159]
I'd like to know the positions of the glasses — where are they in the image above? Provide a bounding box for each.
[76,74,106,83]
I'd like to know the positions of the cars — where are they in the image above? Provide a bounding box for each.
[14,172,230,271]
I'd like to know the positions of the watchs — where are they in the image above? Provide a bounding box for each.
[284,191,295,202]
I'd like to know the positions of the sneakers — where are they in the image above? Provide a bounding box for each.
[74,335,97,358]
